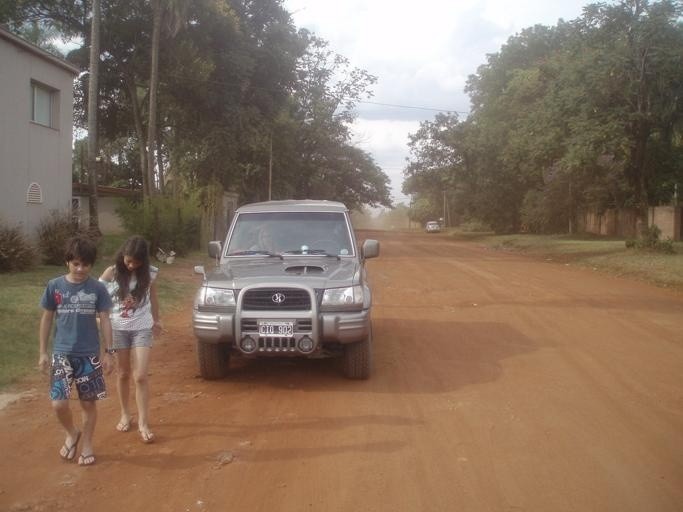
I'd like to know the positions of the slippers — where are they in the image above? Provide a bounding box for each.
[78,455,94,466]
[116,416,133,432]
[137,429,155,444]
[59,430,81,461]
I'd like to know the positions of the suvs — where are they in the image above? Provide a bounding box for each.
[192,199,380,382]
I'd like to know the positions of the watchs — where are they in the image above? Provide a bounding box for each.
[104,347,116,355]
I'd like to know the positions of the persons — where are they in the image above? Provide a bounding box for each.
[96,235,163,446]
[36,237,117,468]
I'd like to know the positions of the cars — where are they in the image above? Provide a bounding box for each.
[424,220,440,233]
